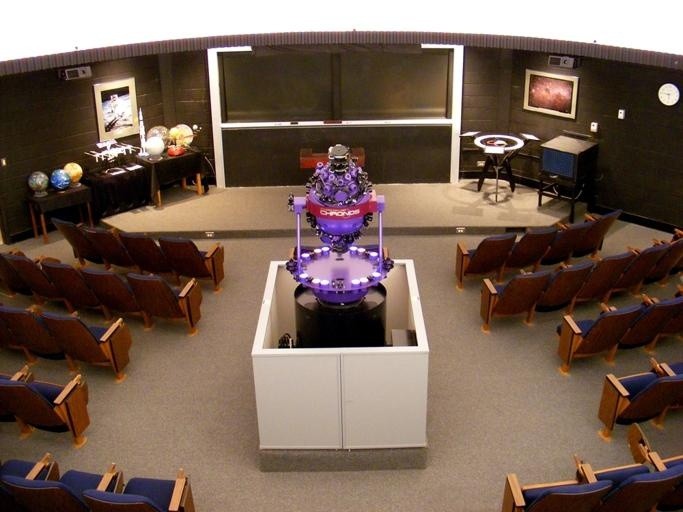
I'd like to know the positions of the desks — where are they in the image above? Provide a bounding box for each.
[534,165,602,226]
[82,161,148,228]
[23,183,97,246]
[135,148,204,208]
[460,129,541,201]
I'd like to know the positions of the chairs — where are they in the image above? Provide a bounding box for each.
[555,284,682,437]
[1,364,91,448]
[457,209,683,334]
[0,248,202,337]
[1,451,196,512]
[500,424,681,511]
[50,217,226,294]
[0,301,132,384]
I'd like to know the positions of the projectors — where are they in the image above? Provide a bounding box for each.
[57,65,93,81]
[548,55,582,69]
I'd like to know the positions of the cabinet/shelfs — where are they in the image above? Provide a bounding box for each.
[537,134,600,225]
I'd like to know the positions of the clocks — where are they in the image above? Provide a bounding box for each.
[656,82,680,107]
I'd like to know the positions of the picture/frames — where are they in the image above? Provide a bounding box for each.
[90,77,141,143]
[522,67,581,120]
